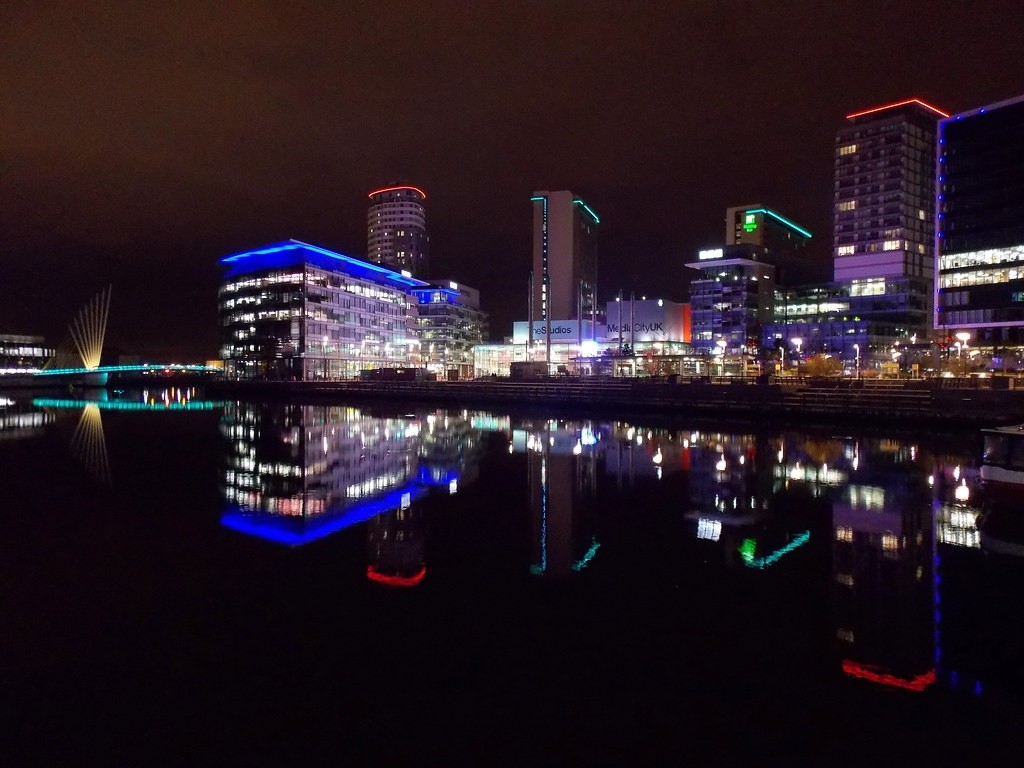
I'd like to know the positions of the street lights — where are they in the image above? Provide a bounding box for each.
[853,343,860,378]
[779,346,784,374]
